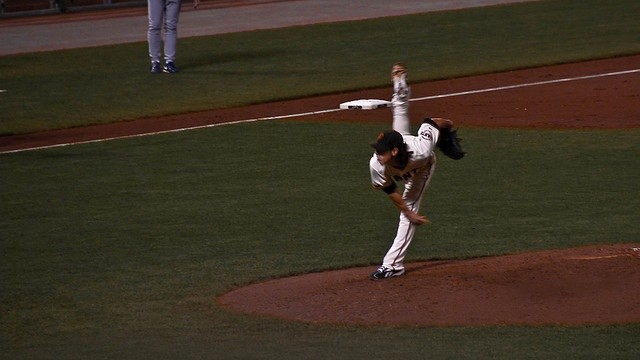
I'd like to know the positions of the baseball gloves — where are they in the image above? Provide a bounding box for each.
[439,136,467,159]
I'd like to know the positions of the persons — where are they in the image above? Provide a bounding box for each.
[368,64,455,280]
[146,1,182,75]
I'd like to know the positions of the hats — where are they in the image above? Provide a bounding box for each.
[369,129,403,153]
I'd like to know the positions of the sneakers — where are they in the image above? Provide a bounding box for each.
[392,61,410,109]
[162,60,177,73]
[367,265,405,281]
[150,60,161,73]
